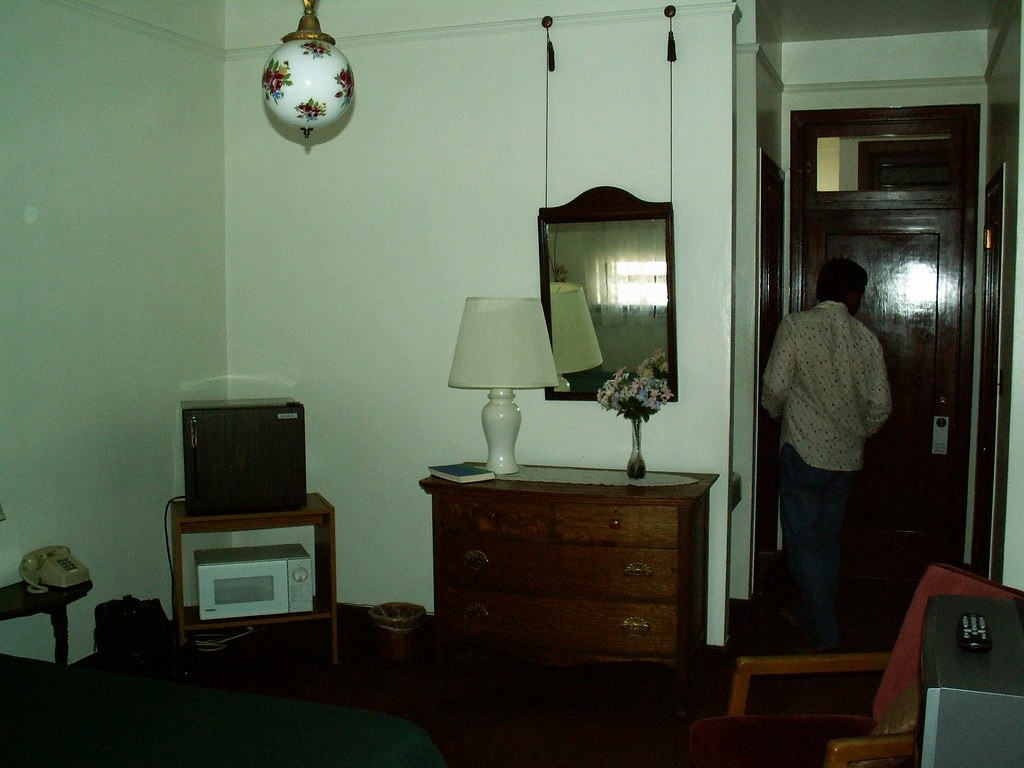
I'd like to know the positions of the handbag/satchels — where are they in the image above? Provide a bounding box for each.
[93,596,175,665]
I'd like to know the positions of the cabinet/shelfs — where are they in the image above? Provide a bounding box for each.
[419,460,720,680]
[170,492,339,664]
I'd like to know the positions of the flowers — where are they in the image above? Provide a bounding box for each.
[598,368,674,461]
[639,347,670,389]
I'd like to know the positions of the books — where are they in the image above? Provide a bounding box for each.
[429,464,495,484]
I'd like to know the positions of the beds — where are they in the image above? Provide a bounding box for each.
[0,652,447,768]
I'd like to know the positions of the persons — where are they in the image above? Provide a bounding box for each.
[760,258,892,650]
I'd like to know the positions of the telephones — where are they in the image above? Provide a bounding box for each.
[21,545,91,588]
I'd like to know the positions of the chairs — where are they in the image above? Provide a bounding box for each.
[688,562,1024,768]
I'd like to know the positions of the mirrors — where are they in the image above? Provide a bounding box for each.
[537,185,678,403]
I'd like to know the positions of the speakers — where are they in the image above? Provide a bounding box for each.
[181,396,306,516]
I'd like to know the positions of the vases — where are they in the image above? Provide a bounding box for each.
[626,419,647,480]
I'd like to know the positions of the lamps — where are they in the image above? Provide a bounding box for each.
[262,0,355,139]
[550,281,602,393]
[447,297,559,475]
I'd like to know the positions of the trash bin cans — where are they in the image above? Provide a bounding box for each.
[368,601,427,662]
